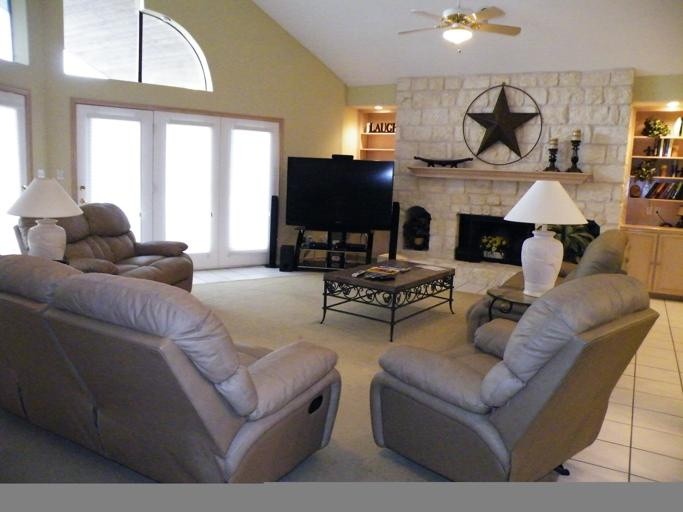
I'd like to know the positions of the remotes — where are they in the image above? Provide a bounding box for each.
[351,270,366,278]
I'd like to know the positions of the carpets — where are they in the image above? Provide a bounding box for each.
[0,272,486,483]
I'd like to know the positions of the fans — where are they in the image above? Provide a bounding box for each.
[399,1,521,44]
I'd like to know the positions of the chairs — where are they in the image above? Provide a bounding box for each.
[369,274,659,482]
[13,203,193,293]
[466,230,629,344]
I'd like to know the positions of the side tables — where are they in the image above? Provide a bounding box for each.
[487,288,538,321]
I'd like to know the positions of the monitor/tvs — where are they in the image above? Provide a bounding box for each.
[285,157,395,231]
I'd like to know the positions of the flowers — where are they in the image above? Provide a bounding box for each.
[479,235,507,253]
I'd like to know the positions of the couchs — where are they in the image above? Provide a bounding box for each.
[0,254,341,483]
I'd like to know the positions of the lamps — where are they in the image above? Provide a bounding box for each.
[503,180,588,298]
[441,7,474,45]
[7,179,84,261]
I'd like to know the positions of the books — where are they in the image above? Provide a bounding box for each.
[645,139,683,199]
[365,265,410,279]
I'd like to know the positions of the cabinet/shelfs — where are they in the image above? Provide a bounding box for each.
[294,227,374,268]
[619,102,683,302]
[358,111,396,160]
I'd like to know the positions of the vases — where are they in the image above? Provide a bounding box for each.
[484,251,506,263]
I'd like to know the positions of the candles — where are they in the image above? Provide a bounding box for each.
[572,128,581,140]
[550,138,557,149]
